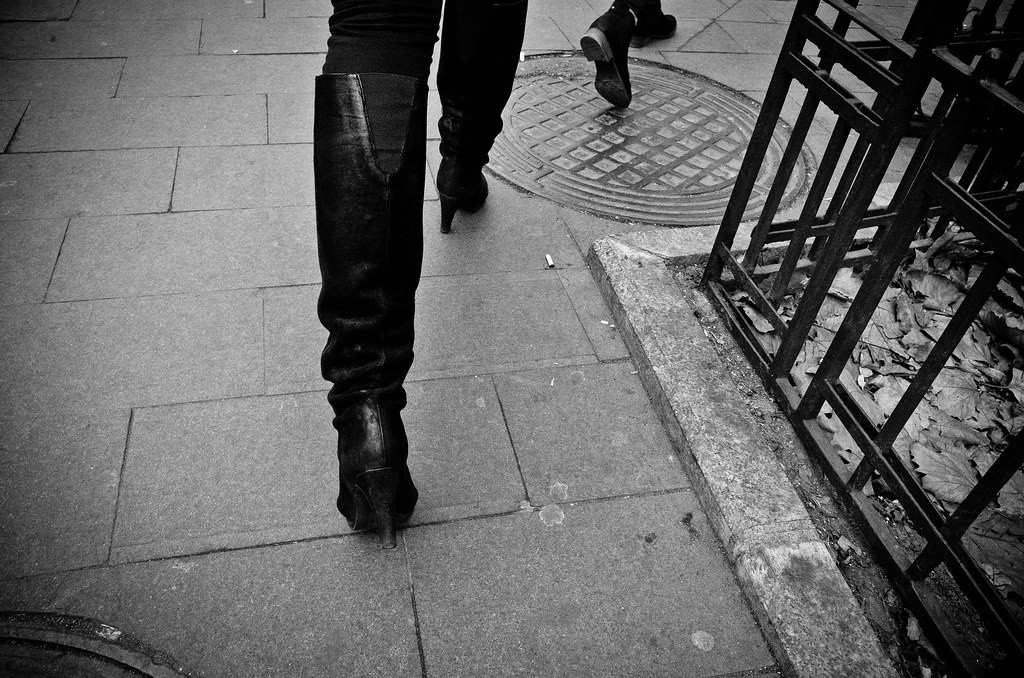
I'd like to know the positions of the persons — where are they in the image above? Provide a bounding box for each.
[580,0,678,108]
[310,1,529,549]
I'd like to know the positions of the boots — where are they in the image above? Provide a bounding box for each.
[314,72,430,548]
[631,0,677,47]
[437,0,528,233]
[580,1,636,108]
